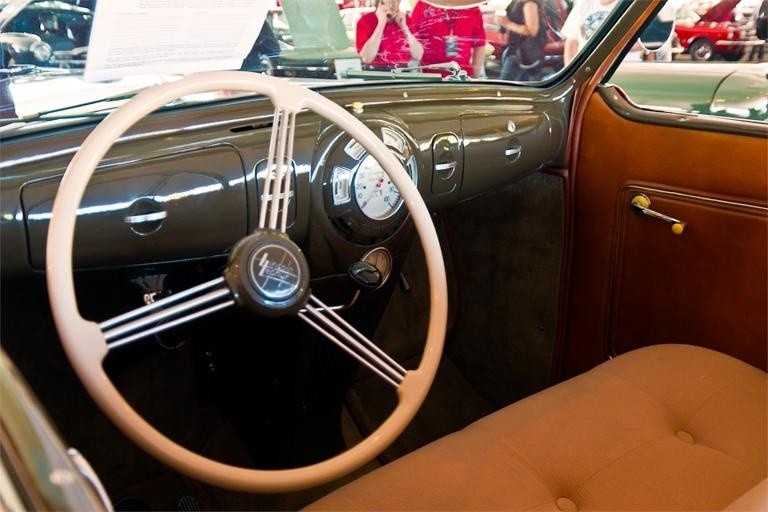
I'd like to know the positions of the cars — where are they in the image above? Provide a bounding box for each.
[0,0,95,75]
[641,1,744,63]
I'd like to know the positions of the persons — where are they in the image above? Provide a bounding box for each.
[0,0,97,70]
[268,2,768,84]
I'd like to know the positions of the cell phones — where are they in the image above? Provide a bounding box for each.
[382,2,393,19]
